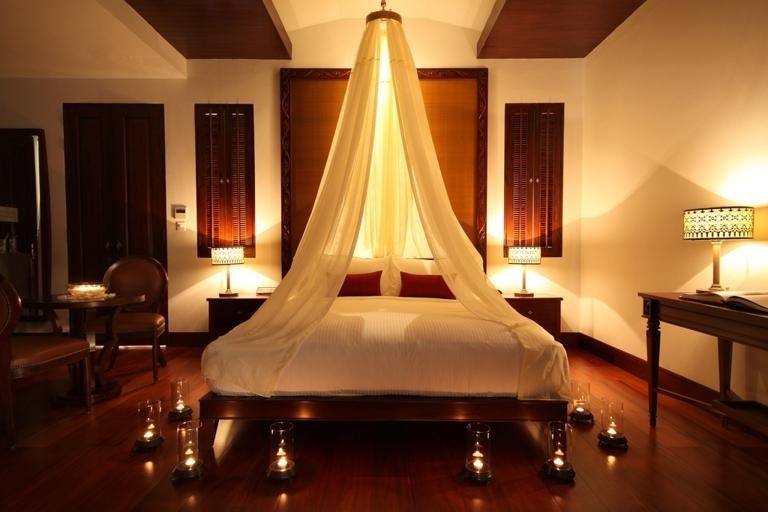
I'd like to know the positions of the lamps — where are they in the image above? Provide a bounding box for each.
[683,207,754,292]
[598,398,628,453]
[508,246,541,297]
[265,422,296,482]
[211,246,245,296]
[133,399,163,452]
[537,420,574,482]
[168,378,193,421]
[568,375,595,427]
[171,420,208,486]
[458,421,493,482]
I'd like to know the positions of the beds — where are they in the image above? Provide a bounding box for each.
[198,257,569,449]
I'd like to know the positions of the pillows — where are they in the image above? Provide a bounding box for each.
[398,271,456,299]
[326,270,383,296]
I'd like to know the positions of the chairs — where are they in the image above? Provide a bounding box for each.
[80,255,167,385]
[0,275,92,447]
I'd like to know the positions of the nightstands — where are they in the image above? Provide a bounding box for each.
[501,292,563,345]
[207,295,268,345]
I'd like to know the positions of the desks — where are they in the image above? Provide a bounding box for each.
[638,292,767,428]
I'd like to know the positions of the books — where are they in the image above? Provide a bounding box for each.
[679,290,767,314]
